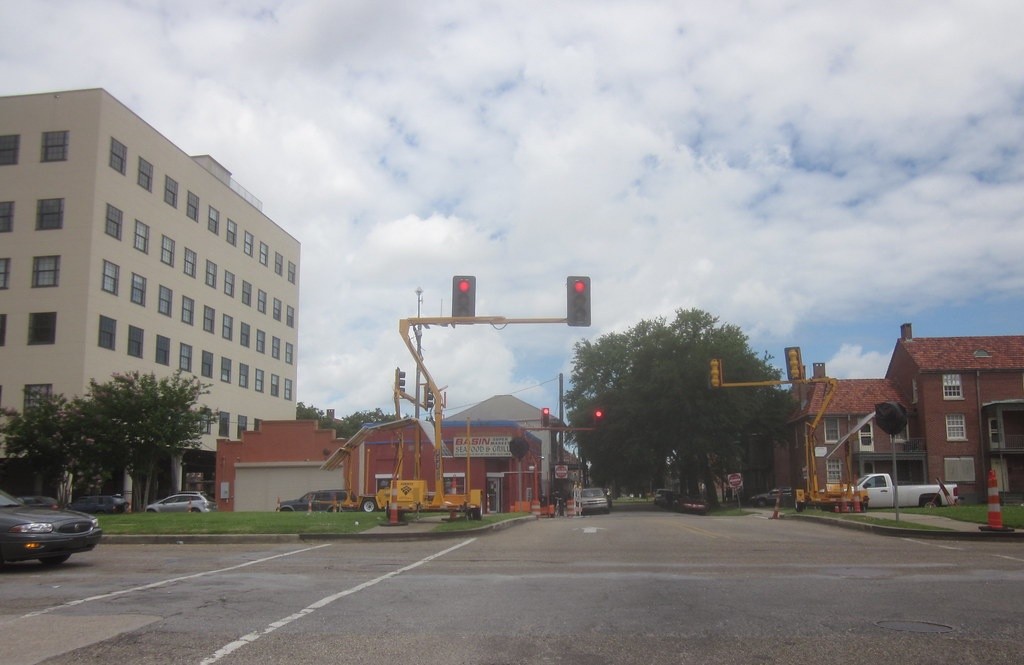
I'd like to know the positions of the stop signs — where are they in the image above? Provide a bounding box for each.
[728,472,744,488]
[554,465,568,480]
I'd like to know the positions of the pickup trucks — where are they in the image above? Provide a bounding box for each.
[845,473,960,512]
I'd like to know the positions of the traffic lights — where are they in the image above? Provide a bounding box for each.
[399,372,406,398]
[566,275,592,327]
[450,274,476,325]
[422,384,435,410]
[591,408,606,423]
[542,408,549,427]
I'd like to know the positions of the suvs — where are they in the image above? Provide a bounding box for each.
[751,485,794,509]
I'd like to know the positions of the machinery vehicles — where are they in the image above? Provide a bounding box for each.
[784,378,870,514]
[357,429,484,524]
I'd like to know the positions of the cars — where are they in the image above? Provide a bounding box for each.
[598,487,612,506]
[673,495,709,515]
[0,487,106,570]
[276,490,357,514]
[63,493,128,514]
[655,489,676,508]
[578,487,610,514]
[15,495,57,512]
[145,489,216,517]
